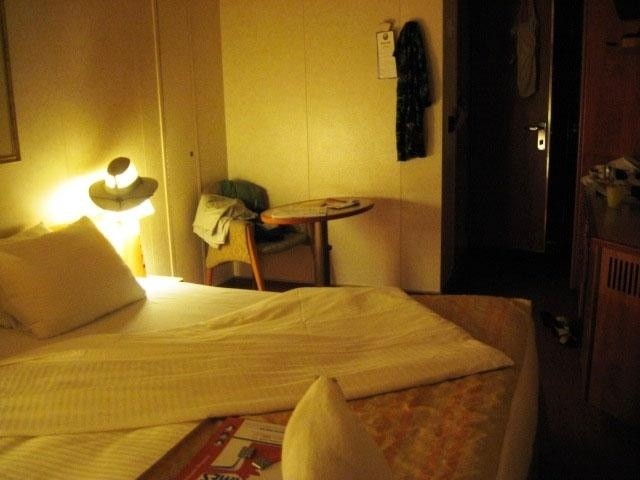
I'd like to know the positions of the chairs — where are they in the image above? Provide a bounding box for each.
[197,180,311,290]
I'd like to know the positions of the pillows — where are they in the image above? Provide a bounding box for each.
[0,213,151,339]
[279,374,394,480]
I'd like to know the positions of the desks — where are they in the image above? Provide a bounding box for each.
[260,196,377,288]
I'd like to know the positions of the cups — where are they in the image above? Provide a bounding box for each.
[605,184,625,209]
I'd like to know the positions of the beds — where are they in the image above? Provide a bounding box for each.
[0,275,542,480]
